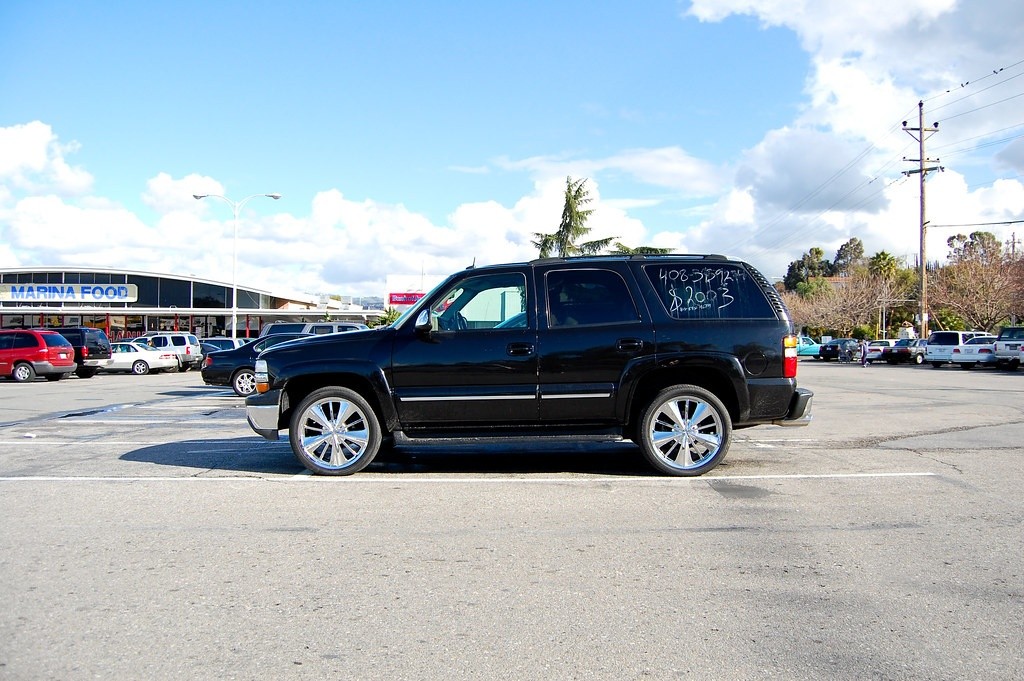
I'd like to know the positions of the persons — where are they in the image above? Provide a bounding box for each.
[858,339,870,367]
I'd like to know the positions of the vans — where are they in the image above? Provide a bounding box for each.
[197,338,246,351]
[260,322,370,337]
[925,331,992,368]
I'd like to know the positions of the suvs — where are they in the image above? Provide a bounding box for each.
[0,329,77,383]
[243,255,813,476]
[993,326,1024,370]
[31,326,114,380]
[128,334,204,373]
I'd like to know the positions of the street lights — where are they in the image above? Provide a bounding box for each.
[194,192,281,337]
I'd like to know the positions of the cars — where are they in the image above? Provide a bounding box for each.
[191,343,223,370]
[952,335,1016,366]
[882,339,930,364]
[202,332,321,398]
[818,338,867,360]
[795,337,825,359]
[98,341,179,374]
[864,340,901,364]
